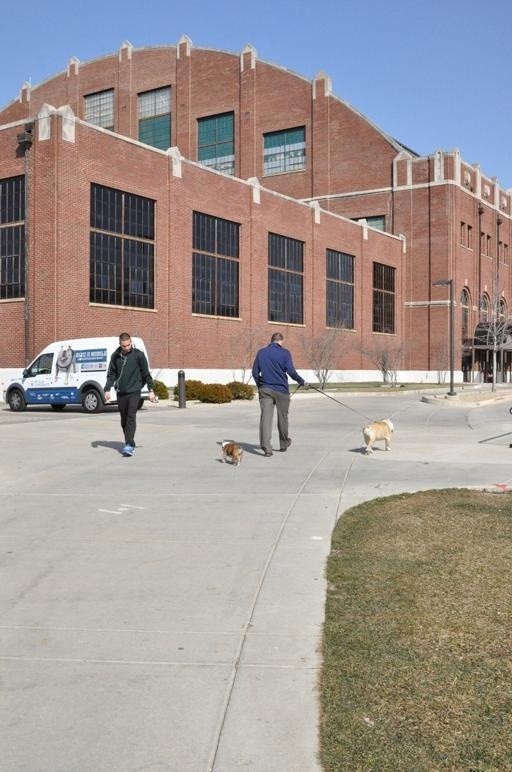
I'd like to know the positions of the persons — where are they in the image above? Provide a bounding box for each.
[104,333,156,456]
[252,333,308,457]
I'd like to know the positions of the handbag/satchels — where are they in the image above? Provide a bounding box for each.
[150,389,154,392]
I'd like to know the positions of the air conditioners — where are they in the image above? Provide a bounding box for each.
[222,442,243,467]
[363,418,393,456]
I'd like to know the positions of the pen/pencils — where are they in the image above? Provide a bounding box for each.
[3,336,151,413]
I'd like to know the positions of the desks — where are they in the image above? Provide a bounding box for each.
[432,279,457,396]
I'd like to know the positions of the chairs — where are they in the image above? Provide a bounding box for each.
[121,444,135,456]
[260,443,273,457]
[280,438,292,452]
